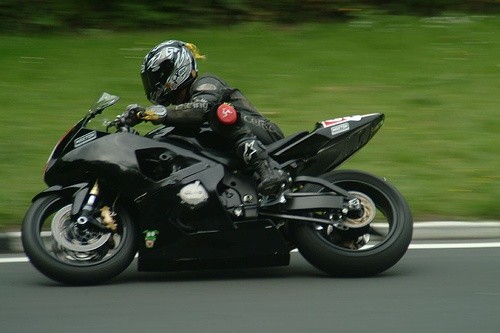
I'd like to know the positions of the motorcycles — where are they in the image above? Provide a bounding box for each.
[21,91,413,283]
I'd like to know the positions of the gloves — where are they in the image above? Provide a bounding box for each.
[123,104,147,127]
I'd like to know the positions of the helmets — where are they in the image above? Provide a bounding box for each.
[140,39,199,106]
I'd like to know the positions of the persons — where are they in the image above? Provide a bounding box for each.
[115,39,294,273]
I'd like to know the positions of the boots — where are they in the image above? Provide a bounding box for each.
[234,135,293,196]
